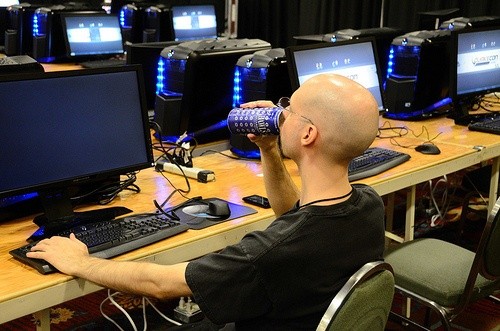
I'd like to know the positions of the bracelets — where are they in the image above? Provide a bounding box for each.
[281,185,355,216]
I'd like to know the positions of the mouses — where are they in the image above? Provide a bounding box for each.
[208,200,231,219]
[416,142,441,155]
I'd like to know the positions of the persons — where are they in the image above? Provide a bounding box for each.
[27,73,388,331]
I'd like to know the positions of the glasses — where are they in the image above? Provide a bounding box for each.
[277,97,314,126]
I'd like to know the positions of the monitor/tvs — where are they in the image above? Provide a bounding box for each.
[450,28,500,126]
[288,36,389,113]
[170,4,219,41]
[0,64,155,242]
[60,12,125,58]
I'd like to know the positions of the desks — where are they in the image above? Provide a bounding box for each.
[0,92,500,331]
[0,50,124,77]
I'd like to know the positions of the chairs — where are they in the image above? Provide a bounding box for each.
[315,261,396,331]
[382,196,500,331]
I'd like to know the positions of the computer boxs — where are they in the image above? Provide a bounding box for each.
[380,29,452,121]
[121,4,171,43]
[31,4,100,62]
[440,16,500,32]
[229,48,292,160]
[7,3,44,55]
[153,37,271,145]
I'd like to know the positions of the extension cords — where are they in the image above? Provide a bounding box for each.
[158,158,215,182]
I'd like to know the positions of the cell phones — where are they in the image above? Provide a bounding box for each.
[243,195,270,208]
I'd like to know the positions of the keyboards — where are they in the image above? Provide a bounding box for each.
[468,111,500,135]
[8,212,188,276]
[347,146,411,182]
